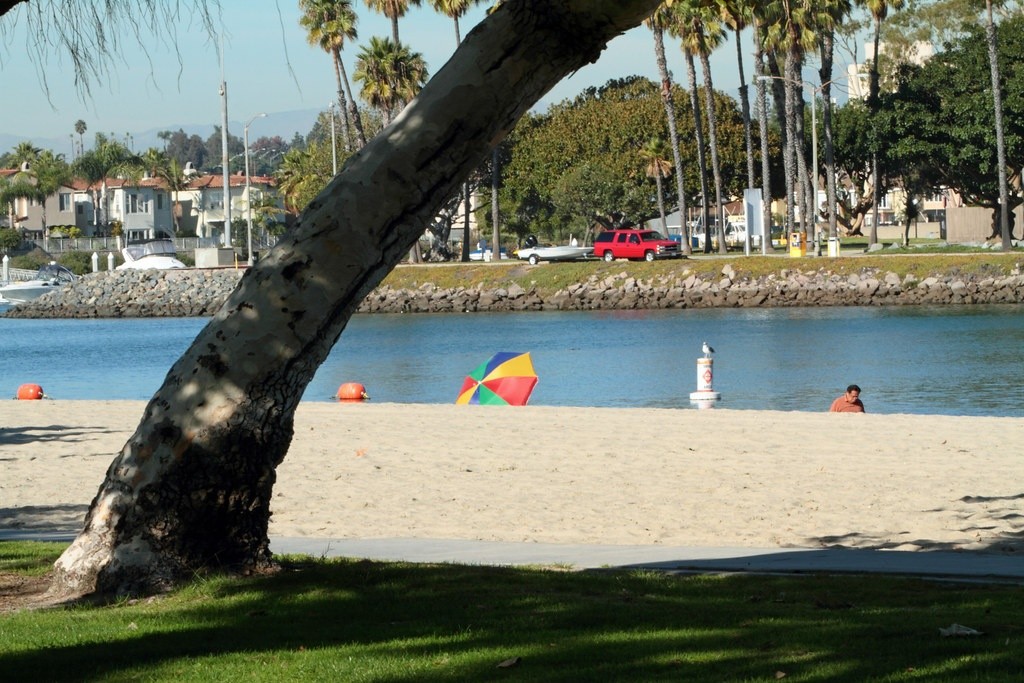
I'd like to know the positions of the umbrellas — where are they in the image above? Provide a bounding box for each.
[456,351,539,406]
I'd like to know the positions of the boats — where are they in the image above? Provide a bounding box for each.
[516,235,594,261]
[113,238,186,269]
[469,246,510,261]
[0,261,83,301]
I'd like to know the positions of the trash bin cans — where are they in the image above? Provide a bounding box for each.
[828,237,840,257]
[790,232,806,257]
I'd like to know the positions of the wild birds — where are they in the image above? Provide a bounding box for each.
[701,341,716,357]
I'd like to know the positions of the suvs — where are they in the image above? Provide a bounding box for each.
[592,230,683,261]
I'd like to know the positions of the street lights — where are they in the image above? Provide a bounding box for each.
[246,113,270,265]
[757,72,870,255]
[329,100,338,176]
[218,78,232,249]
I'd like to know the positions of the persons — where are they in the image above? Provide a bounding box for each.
[829,385,865,413]
